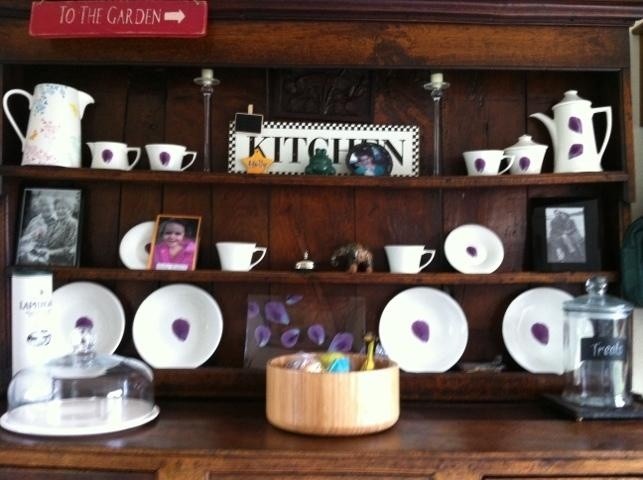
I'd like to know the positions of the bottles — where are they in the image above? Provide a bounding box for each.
[561,274,637,411]
[304,148,337,176]
[293,251,315,270]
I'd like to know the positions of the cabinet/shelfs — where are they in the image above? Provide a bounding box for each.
[0,1,643,479]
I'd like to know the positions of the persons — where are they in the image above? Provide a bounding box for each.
[556,212,581,255]
[30,192,78,267]
[544,207,566,264]
[150,218,196,271]
[18,194,58,266]
[353,148,384,176]
[28,196,41,216]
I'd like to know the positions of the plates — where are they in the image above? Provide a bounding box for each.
[443,223,505,276]
[1,396,162,440]
[117,220,158,271]
[52,282,127,358]
[377,286,472,376]
[501,287,594,375]
[131,283,225,370]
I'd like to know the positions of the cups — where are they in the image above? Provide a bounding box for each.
[383,244,437,275]
[462,149,515,178]
[213,241,269,274]
[145,143,198,172]
[85,141,143,173]
[504,132,549,174]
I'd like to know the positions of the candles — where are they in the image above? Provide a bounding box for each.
[429,72,444,82]
[201,68,213,79]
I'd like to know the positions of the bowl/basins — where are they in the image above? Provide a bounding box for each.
[262,350,402,439]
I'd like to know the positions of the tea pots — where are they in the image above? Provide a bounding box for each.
[2,81,96,170]
[528,89,612,175]
